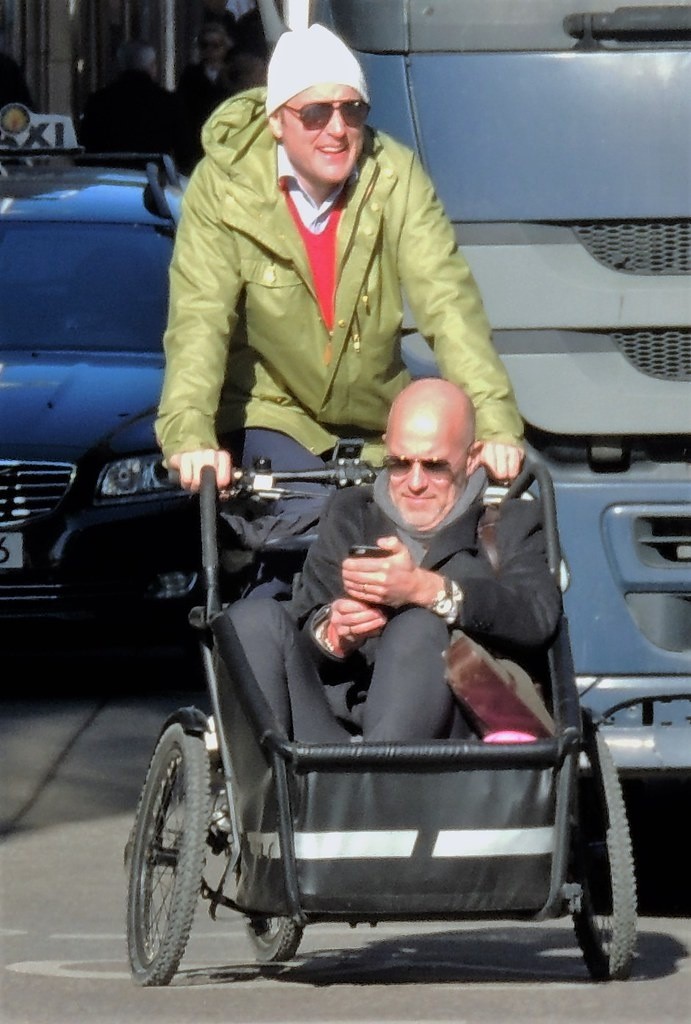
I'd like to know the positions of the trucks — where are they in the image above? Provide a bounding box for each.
[256,0,691,780]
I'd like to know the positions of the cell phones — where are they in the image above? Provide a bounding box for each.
[348,544,392,559]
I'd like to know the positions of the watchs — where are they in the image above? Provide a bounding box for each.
[430,574,453,616]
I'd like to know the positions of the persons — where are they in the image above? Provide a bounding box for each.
[295,373,561,742]
[155,23,524,546]
[79,0,266,173]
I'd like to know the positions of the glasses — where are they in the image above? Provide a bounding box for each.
[383,439,474,478]
[281,101,371,130]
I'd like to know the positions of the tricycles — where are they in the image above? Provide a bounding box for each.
[124,438,638,987]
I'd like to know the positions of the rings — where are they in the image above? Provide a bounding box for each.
[363,584,366,593]
[349,626,352,634]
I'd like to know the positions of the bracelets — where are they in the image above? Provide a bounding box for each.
[323,622,333,652]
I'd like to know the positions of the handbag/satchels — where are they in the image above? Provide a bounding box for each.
[440,623,557,740]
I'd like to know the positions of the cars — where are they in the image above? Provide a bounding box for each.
[0,153,189,626]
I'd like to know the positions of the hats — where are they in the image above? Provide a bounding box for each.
[265,23,370,117]
